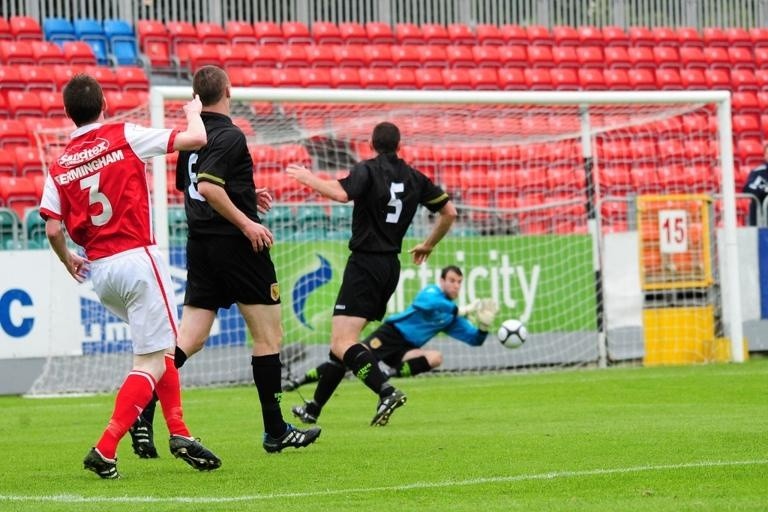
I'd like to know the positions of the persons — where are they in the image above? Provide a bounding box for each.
[281,265,500,392]
[286,121,458,427]
[37,73,221,480]
[741,146,768,226]
[126,64,322,459]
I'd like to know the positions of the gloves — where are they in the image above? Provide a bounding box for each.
[477,299,500,326]
[459,299,481,317]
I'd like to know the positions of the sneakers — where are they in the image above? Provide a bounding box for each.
[282,378,299,393]
[369,387,408,426]
[128,413,161,460]
[261,422,324,454]
[82,446,121,481]
[291,401,321,424]
[167,433,223,472]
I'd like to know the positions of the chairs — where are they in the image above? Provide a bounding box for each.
[0,90,76,249]
[144,156,183,235]
[233,21,766,166]
[255,145,353,238]
[419,167,754,237]
[1,18,239,89]
[103,90,190,131]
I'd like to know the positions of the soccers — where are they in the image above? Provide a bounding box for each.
[498,319,527,348]
